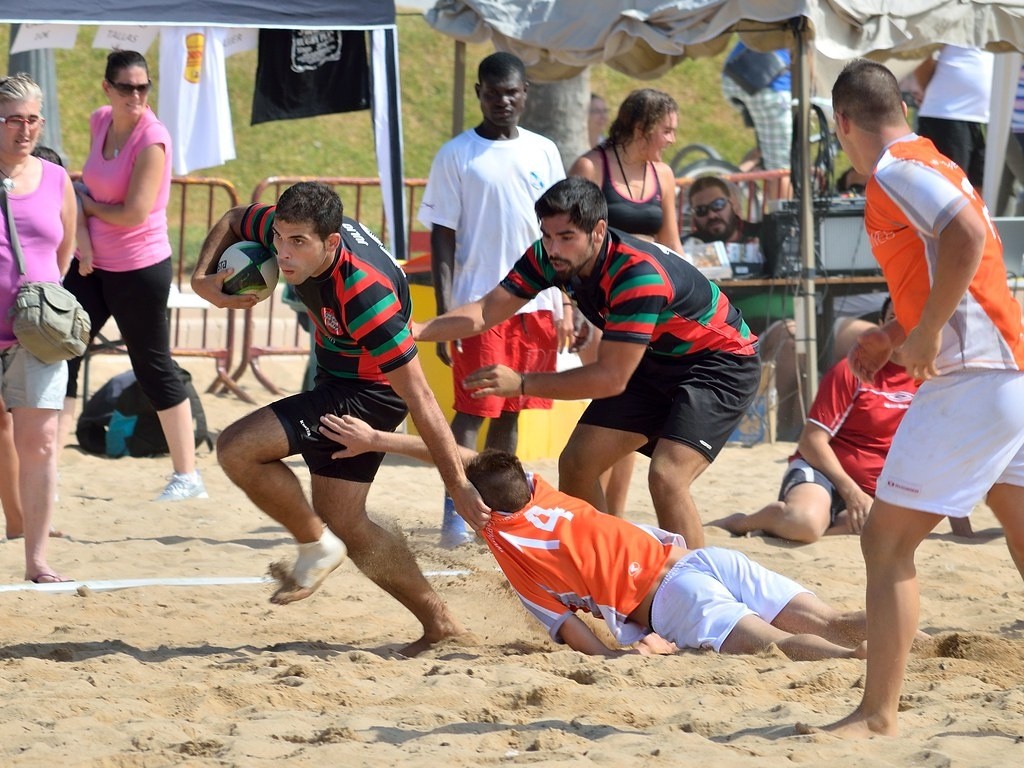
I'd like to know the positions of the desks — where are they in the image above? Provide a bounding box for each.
[697,276,892,377]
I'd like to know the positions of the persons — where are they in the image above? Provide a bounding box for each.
[412,173,761,549]
[913,44,994,174]
[418,52,594,543]
[32,147,95,276]
[724,41,794,213]
[795,59,1024,737]
[681,178,884,436]
[191,182,491,652]
[589,93,609,147]
[319,412,929,660]
[1,75,78,584]
[67,49,210,504]
[703,295,978,543]
[557,91,684,367]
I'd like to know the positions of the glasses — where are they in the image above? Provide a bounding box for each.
[846,183,867,194]
[107,79,152,97]
[0,115,44,130]
[695,197,729,219]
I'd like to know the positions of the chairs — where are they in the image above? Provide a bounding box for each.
[667,142,763,239]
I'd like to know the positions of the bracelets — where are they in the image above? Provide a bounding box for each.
[521,374,525,395]
[564,303,572,305]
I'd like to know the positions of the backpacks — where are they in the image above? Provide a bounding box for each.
[74,361,214,460]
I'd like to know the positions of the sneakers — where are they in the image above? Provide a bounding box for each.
[155,470,209,502]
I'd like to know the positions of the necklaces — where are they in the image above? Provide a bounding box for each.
[614,146,648,200]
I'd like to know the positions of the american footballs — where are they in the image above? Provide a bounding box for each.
[217,240,280,304]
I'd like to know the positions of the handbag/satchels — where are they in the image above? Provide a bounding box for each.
[10,281,93,363]
[723,46,789,97]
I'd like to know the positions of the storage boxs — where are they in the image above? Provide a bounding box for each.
[724,361,794,445]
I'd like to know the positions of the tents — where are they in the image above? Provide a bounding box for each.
[0,1,405,263]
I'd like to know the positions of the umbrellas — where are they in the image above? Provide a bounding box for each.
[422,1,1024,412]
